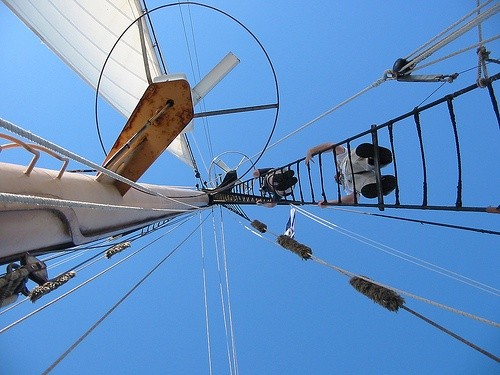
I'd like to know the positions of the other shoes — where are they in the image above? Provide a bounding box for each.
[271,170,294,182]
[354,143,392,165]
[275,178,298,191]
[359,173,395,198]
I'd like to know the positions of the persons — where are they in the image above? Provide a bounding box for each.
[252,167,298,209]
[304,142,398,209]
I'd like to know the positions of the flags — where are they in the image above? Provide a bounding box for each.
[284,207,296,239]
